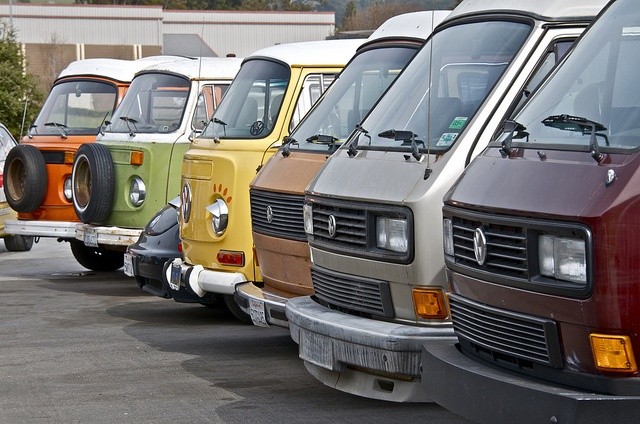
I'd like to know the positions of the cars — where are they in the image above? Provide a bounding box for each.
[124,195,199,303]
[0,122,33,252]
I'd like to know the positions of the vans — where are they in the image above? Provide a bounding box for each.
[162,38,366,324]
[3,58,142,271]
[71,55,245,246]
[421,0,636,422]
[286,0,609,405]
[234,9,451,330]
